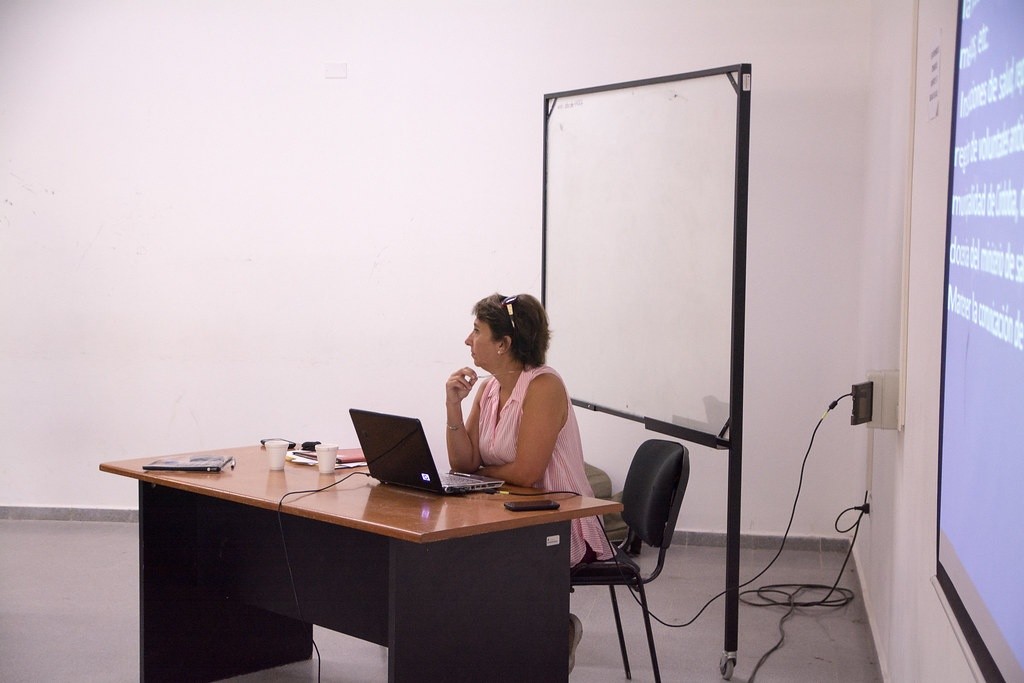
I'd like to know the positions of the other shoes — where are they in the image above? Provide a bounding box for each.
[570,614,582,673]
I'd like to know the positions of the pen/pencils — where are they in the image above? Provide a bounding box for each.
[230,457,235,470]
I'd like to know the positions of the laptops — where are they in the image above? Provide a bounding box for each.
[349,409,505,492]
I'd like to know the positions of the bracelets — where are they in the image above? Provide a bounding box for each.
[446,422,464,430]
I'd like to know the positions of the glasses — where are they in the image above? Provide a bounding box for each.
[503,295,516,329]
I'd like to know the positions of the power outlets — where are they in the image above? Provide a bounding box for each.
[868,494,873,518]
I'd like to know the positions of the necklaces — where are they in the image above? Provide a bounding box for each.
[469,368,521,379]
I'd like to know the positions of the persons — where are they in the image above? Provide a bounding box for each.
[444,293,618,674]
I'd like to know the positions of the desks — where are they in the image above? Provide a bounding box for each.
[100,443,624,683]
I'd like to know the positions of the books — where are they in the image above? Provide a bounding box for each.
[292,443,366,464]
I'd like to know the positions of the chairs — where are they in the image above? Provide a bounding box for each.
[571,438,690,683]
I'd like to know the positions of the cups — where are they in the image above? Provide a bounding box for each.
[265,440,289,471]
[315,444,338,473]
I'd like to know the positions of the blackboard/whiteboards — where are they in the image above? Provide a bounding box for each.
[529,62,752,452]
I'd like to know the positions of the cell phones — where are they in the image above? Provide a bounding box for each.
[261,438,296,448]
[504,499,560,511]
